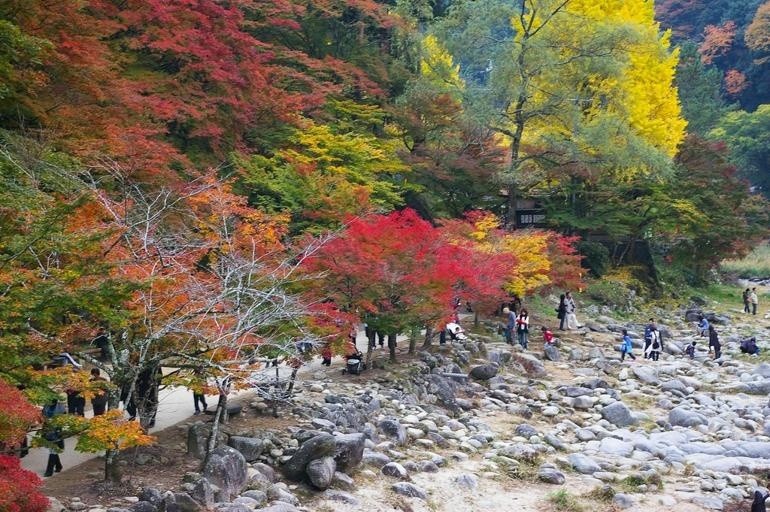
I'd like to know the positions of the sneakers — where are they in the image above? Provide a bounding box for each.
[194,409,200,415]
[204,404,207,411]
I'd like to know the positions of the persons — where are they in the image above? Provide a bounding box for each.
[187,368,208,415]
[243,302,386,367]
[751,490,770,512]
[643,318,657,359]
[697,315,710,338]
[685,340,697,360]
[0,348,164,476]
[707,325,722,361]
[499,289,583,353]
[438,297,475,344]
[618,329,636,363]
[743,288,751,313]
[750,287,759,315]
[649,323,663,361]
[748,340,761,355]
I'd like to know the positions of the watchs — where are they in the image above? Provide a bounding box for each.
[739,338,757,353]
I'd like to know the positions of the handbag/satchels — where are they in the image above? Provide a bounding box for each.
[626,345,633,354]
[652,342,659,349]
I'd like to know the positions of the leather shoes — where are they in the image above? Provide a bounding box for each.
[44,471,53,477]
[55,464,62,472]
